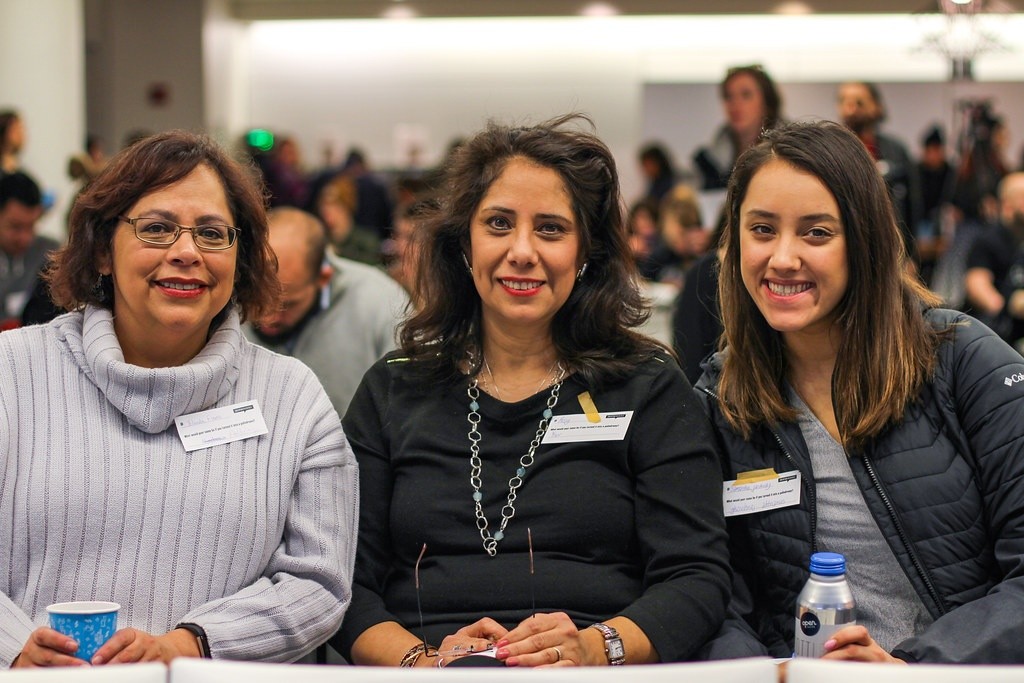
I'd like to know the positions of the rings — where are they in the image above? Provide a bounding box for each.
[438,658,446,667]
[555,647,562,663]
[453,645,462,657]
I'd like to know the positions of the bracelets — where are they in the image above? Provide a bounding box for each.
[177,623,211,660]
[400,643,437,668]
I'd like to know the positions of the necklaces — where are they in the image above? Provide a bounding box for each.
[465,348,565,558]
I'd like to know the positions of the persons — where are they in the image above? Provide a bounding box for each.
[0,62,1024,356]
[344,116,727,668]
[3,129,361,666]
[695,122,1024,670]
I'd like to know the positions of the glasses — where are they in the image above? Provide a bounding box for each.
[728,65,763,75]
[120,216,241,250]
[416,528,535,657]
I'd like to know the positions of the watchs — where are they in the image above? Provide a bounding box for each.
[590,622,626,667]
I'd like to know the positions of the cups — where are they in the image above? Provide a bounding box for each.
[46,601,121,664]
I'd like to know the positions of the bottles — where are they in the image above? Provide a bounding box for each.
[793,552,856,660]
